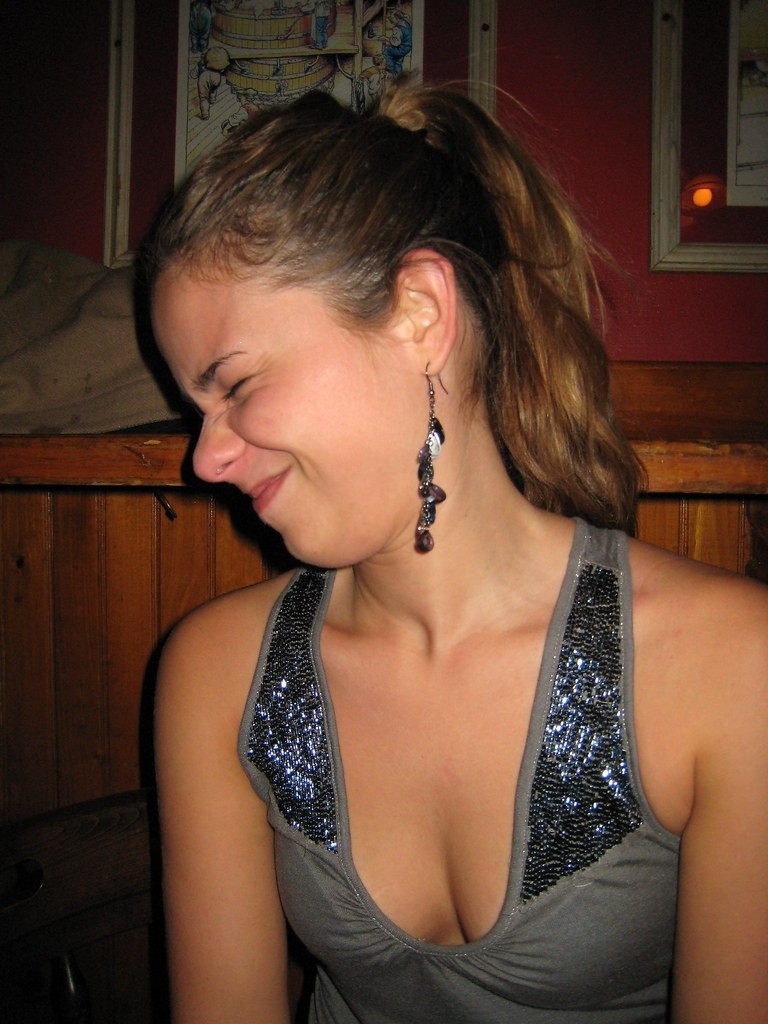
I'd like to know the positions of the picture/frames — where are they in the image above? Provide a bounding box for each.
[101,0,497,275]
[650,0,768,275]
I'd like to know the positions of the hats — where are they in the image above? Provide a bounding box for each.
[0,237,204,438]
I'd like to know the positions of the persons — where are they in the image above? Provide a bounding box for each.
[146,77,768,1022]
